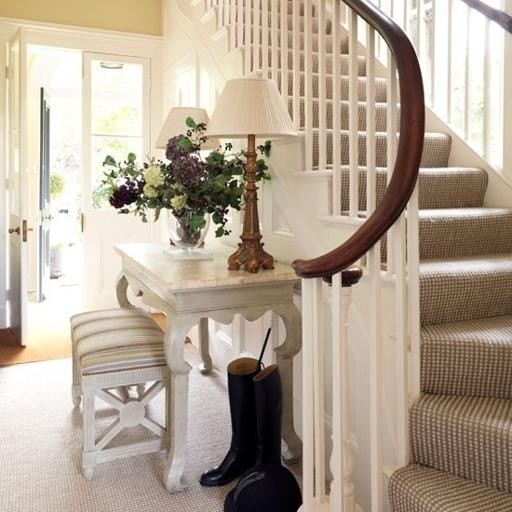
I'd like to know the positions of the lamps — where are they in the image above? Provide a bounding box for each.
[155,77,299,272]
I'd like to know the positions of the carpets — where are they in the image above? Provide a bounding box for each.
[1,341,302,512]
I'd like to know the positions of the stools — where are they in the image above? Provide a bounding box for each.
[70,307,191,482]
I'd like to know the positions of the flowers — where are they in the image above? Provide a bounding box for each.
[93,117,272,244]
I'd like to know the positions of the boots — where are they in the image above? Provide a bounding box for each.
[200,357,282,487]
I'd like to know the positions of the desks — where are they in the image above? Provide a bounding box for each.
[111,247,301,493]
